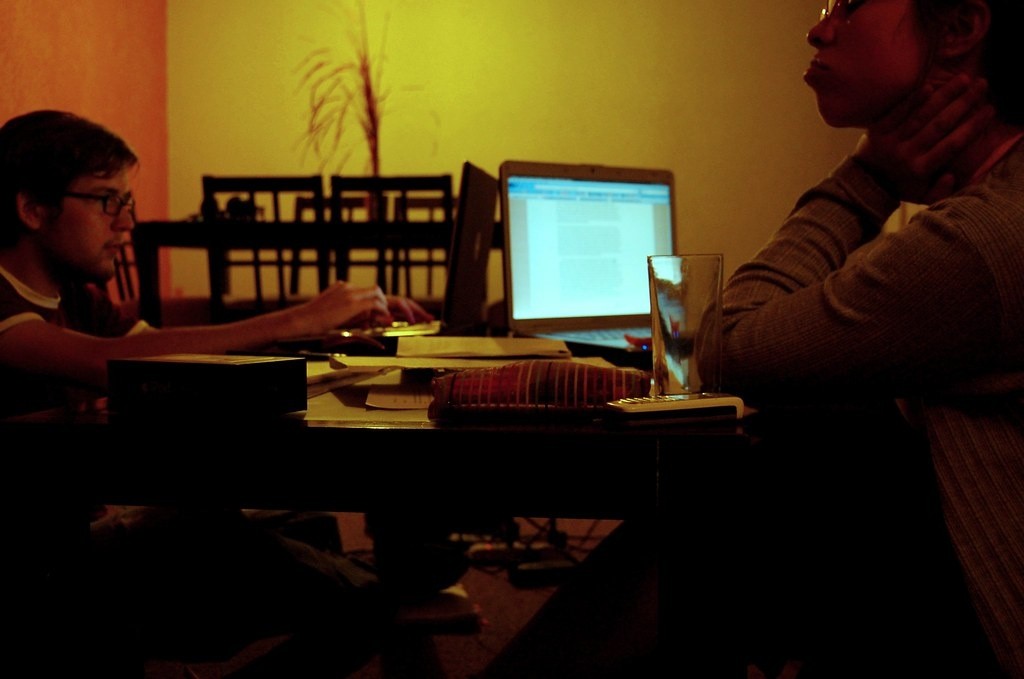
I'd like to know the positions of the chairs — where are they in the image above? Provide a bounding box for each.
[203,175,457,302]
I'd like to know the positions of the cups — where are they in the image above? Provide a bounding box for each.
[647,253,723,399]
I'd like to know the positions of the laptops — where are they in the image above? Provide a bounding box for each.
[499,159,678,368]
[280,161,499,346]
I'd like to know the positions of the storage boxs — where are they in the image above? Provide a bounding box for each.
[107,355,307,414]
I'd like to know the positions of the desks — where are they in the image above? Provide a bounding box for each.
[0,325,756,679]
[130,219,507,325]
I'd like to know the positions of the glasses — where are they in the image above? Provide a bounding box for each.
[54,193,134,216]
[817,0,869,26]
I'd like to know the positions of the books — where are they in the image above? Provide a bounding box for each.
[396,337,572,359]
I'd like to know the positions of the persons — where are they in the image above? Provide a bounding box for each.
[0,110,434,679]
[470,0,1024,679]
